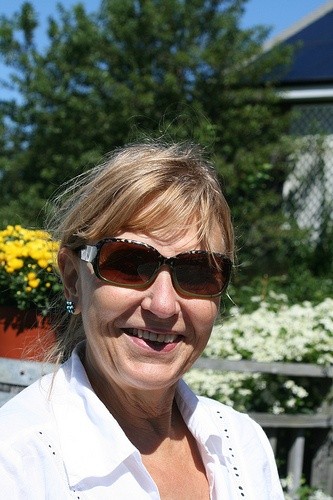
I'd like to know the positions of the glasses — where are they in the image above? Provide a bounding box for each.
[71,239,235,298]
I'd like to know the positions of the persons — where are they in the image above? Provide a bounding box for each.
[0,137,287,500]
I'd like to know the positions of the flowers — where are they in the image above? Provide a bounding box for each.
[0,225,63,293]
[182,292,333,416]
